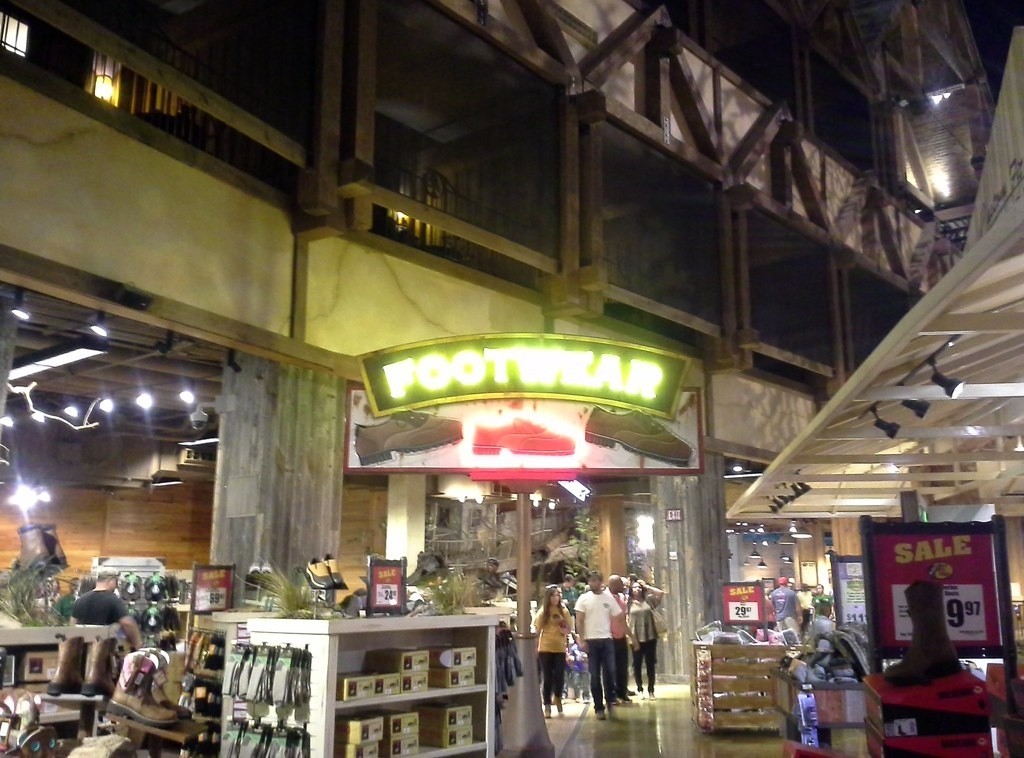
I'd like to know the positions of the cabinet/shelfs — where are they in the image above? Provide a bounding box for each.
[219,614,500,758]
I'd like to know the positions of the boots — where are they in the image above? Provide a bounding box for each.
[3,523,68,588]
[81,636,117,696]
[883,580,962,686]
[47,633,82,695]
[106,650,192,728]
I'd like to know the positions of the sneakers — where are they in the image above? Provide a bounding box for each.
[472,417,575,456]
[406,551,441,585]
[354,410,463,466]
[249,562,272,574]
[306,553,343,588]
[499,572,517,590]
[585,404,692,464]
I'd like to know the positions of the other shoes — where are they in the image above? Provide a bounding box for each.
[554,696,562,711]
[545,704,551,718]
[639,691,644,700]
[584,700,589,703]
[649,691,655,700]
[596,690,635,719]
[576,698,580,703]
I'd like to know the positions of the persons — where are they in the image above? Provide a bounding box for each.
[535,571,664,721]
[752,577,836,633]
[69,567,143,650]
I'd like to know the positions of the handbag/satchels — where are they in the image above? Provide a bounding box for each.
[653,612,667,634]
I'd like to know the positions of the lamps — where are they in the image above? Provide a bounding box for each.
[90,310,110,337]
[93,74,113,105]
[226,359,242,373]
[179,379,196,404]
[11,287,31,321]
[153,329,174,358]
[135,384,154,409]
[725,357,966,568]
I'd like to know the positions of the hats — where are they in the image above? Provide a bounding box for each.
[98,569,116,580]
[779,577,787,584]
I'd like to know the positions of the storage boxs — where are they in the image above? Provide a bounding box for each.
[332,646,477,758]
[863,669,995,758]
